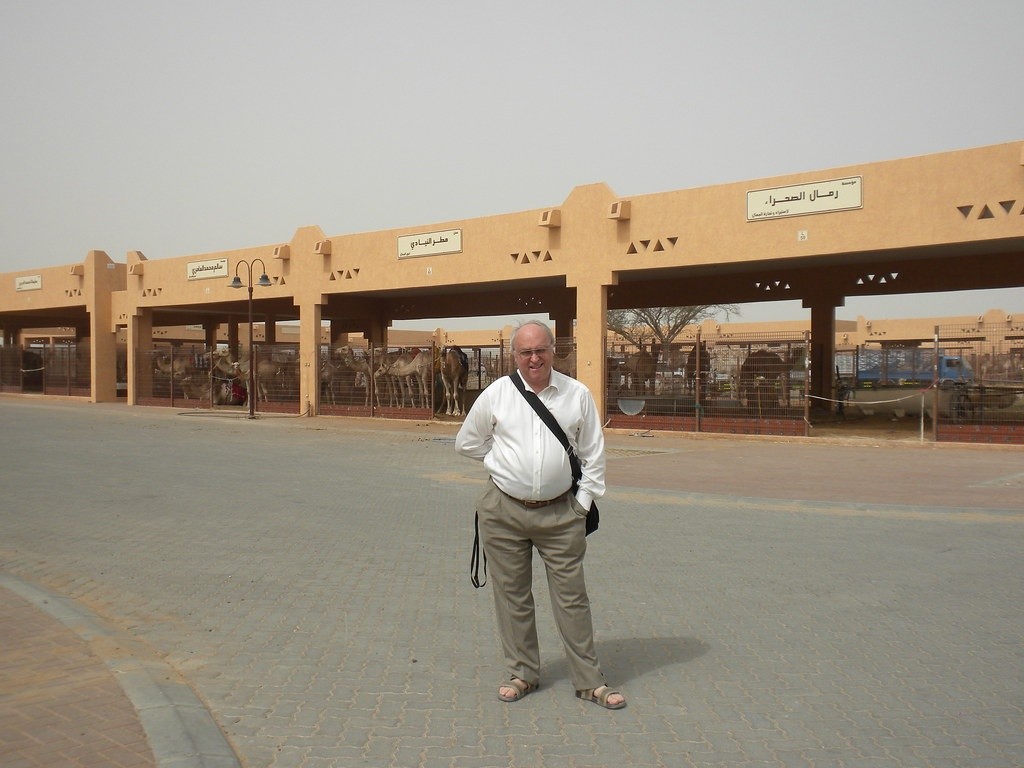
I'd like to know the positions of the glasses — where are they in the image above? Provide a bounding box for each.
[512,345,554,361]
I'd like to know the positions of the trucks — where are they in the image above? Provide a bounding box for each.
[774,371,857,402]
[858,356,974,386]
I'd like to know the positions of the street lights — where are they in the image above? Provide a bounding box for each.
[227,259,273,418]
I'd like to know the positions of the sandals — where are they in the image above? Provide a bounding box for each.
[497,680,540,702]
[575,686,627,709]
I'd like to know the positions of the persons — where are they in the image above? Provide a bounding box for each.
[455,319,626,710]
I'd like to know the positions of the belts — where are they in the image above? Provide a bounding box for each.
[502,489,570,511]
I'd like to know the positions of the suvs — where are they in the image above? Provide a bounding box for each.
[657,362,685,394]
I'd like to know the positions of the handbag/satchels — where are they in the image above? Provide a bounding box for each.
[572,477,599,537]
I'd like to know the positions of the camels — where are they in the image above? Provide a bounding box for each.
[373,346,469,415]
[740,347,803,414]
[282,346,372,408]
[968,350,1024,383]
[552,349,577,379]
[483,353,514,381]
[607,348,685,396]
[686,325,710,399]
[156,350,277,402]
